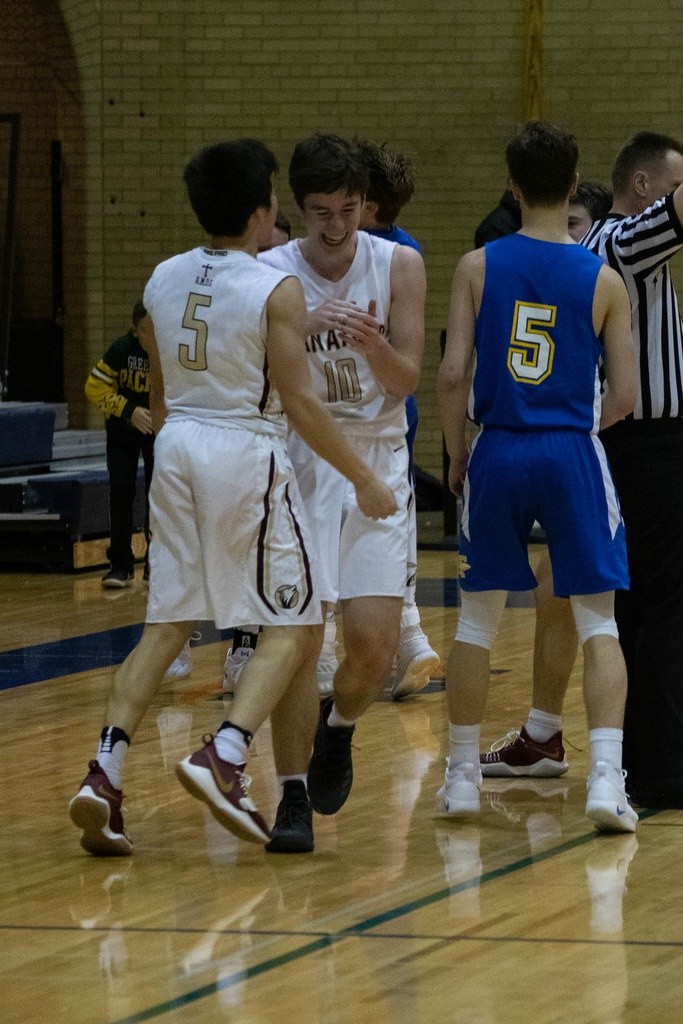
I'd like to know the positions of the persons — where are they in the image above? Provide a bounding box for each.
[84,301,155,589]
[67,697,637,1023]
[478,131,682,807]
[68,138,398,858]
[260,136,428,852]
[162,141,441,698]
[437,117,637,828]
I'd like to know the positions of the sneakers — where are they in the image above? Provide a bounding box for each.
[393,702,442,771]
[479,726,569,777]
[316,640,339,693]
[264,788,315,852]
[143,566,150,586]
[70,858,134,928]
[70,759,134,855]
[438,821,483,870]
[164,631,201,679]
[391,636,441,697]
[101,561,135,587]
[176,733,272,844]
[435,757,484,816]
[308,696,356,816]
[586,761,638,833]
[586,832,640,895]
[223,647,254,694]
[484,777,568,823]
